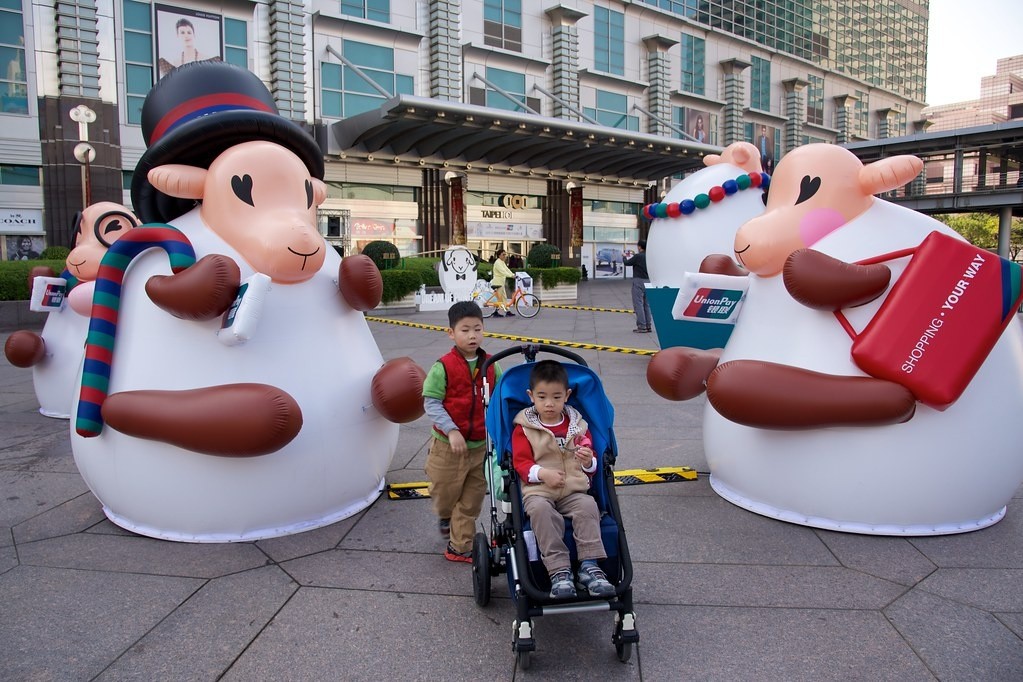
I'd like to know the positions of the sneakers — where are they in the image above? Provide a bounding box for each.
[550,568,577,598]
[438,516,452,539]
[576,565,616,596]
[443,540,473,563]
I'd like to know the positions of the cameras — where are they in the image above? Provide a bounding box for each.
[624,250,630,254]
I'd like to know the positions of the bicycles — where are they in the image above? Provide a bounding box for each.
[472,275,540,319]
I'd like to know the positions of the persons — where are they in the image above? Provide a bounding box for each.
[511,360,616,599]
[693,115,705,143]
[622,240,652,333]
[171,19,207,68]
[490,250,516,317]
[9,235,39,260]
[422,302,504,563]
[757,125,772,174]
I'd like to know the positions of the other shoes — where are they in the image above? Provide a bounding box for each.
[506,311,515,316]
[646,325,651,332]
[494,312,503,317]
[633,327,647,332]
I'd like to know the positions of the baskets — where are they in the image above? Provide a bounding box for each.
[522,277,531,287]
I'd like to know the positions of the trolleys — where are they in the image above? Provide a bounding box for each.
[464,338,643,670]
